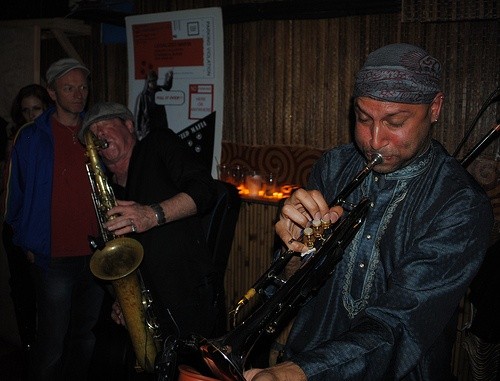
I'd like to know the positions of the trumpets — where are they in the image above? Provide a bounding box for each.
[195,152,384,381]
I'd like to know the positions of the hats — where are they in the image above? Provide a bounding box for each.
[46,58,90,85]
[357,42,445,104]
[144,70,159,93]
[78,102,134,145]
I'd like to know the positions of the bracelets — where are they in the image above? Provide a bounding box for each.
[151,203,166,226]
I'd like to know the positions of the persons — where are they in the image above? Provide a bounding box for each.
[77,102,219,380]
[5,83,57,365]
[134,68,174,141]
[241,43,494,381]
[6,57,116,381]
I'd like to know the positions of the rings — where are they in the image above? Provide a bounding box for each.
[128,218,134,225]
[132,224,136,232]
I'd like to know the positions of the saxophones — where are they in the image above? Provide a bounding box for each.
[78,129,164,378]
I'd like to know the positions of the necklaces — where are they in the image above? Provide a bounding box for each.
[61,123,80,144]
